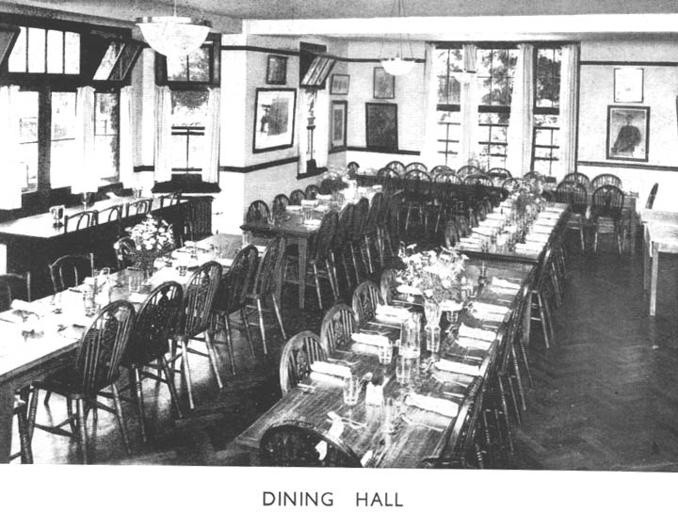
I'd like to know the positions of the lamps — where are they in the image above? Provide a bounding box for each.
[377,0,417,77]
[133,0,214,60]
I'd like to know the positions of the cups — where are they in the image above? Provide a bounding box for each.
[128,275,139,293]
[267,195,347,226]
[396,357,411,382]
[343,375,358,405]
[378,340,393,364]
[424,325,441,352]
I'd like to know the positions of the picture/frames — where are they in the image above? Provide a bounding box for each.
[264,54,290,86]
[250,86,298,155]
[330,99,349,149]
[364,101,400,151]
[611,67,647,104]
[372,66,397,101]
[328,72,352,96]
[603,104,652,163]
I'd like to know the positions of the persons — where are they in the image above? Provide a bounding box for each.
[611,114,642,158]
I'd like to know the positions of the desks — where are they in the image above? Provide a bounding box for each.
[633,196,678,317]
